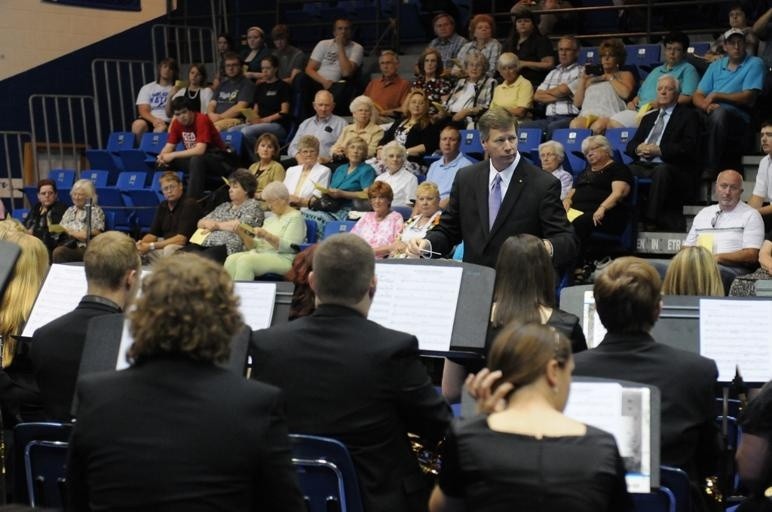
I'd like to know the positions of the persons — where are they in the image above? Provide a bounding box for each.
[65,251,309,512]
[428,320,633,512]
[734,380,772,512]
[32,231,143,425]
[248,232,454,512]
[571,254,718,512]
[0,233,50,507]
[1,0,772,396]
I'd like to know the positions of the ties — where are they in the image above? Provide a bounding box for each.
[648,112,666,146]
[488,173,501,228]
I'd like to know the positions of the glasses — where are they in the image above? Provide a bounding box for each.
[712,210,722,227]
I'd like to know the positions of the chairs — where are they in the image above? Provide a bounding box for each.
[3,375,770,511]
[7,32,771,332]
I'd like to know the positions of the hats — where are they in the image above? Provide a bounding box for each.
[723,29,745,41]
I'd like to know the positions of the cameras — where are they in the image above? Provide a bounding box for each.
[585,57,605,77]
